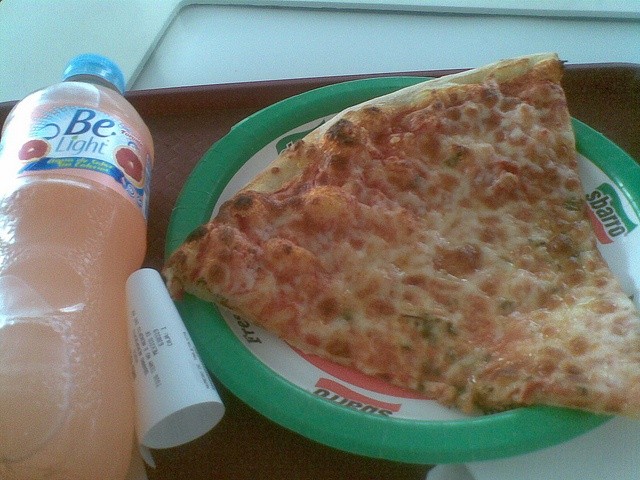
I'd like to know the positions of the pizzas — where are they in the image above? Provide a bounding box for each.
[162,53,640,421]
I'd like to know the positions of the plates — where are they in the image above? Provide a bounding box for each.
[163,80,639,464]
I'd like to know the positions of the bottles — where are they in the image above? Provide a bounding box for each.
[0,54,154,480]
